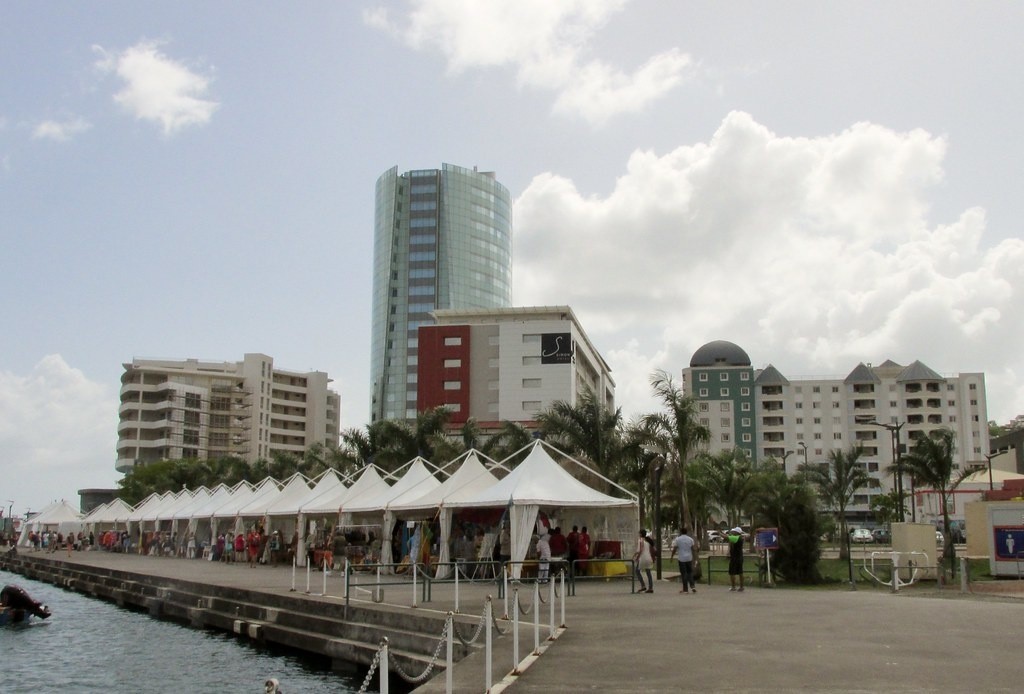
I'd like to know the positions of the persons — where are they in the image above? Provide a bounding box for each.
[565,525,581,578]
[719,528,750,591]
[578,526,591,575]
[4,609,49,633]
[264,677,282,694]
[632,530,656,594]
[0,521,567,584]
[670,529,699,594]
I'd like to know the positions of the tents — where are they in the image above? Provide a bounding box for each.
[439,438,642,581]
[338,455,451,568]
[17,460,410,574]
[383,448,514,579]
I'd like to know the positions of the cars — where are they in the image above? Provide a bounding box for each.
[936,531,945,543]
[850,528,874,544]
[707,530,729,543]
[871,529,890,543]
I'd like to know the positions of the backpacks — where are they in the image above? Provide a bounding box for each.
[251,533,259,546]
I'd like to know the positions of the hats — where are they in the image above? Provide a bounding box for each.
[273,531,278,534]
[731,526,742,534]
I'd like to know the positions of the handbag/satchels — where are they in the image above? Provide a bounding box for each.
[271,537,276,547]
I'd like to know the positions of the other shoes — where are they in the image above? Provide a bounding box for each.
[680,591,688,594]
[737,588,744,591]
[638,588,646,591]
[691,588,696,592]
[729,587,736,591]
[646,589,653,593]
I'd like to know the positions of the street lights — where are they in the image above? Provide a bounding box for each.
[984,449,1007,490]
[865,419,906,524]
[798,441,810,486]
